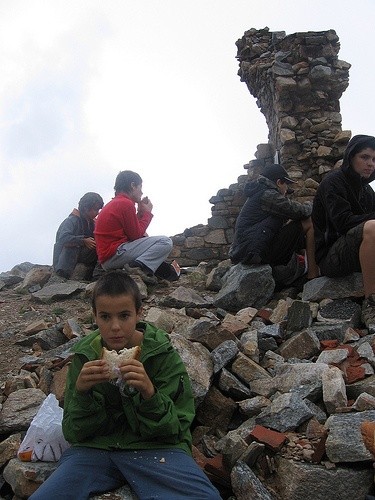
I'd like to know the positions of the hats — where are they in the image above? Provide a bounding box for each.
[262,164,297,184]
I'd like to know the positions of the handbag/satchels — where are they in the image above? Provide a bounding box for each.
[17,394,71,461]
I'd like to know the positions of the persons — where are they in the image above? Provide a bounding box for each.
[93,169,173,283]
[230,162,313,283]
[311,135,375,334]
[53,192,104,277]
[27,269,226,500]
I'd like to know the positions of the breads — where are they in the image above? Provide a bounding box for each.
[101,345,142,380]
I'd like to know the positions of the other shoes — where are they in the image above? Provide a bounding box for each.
[360,294,374,332]
[120,261,158,284]
